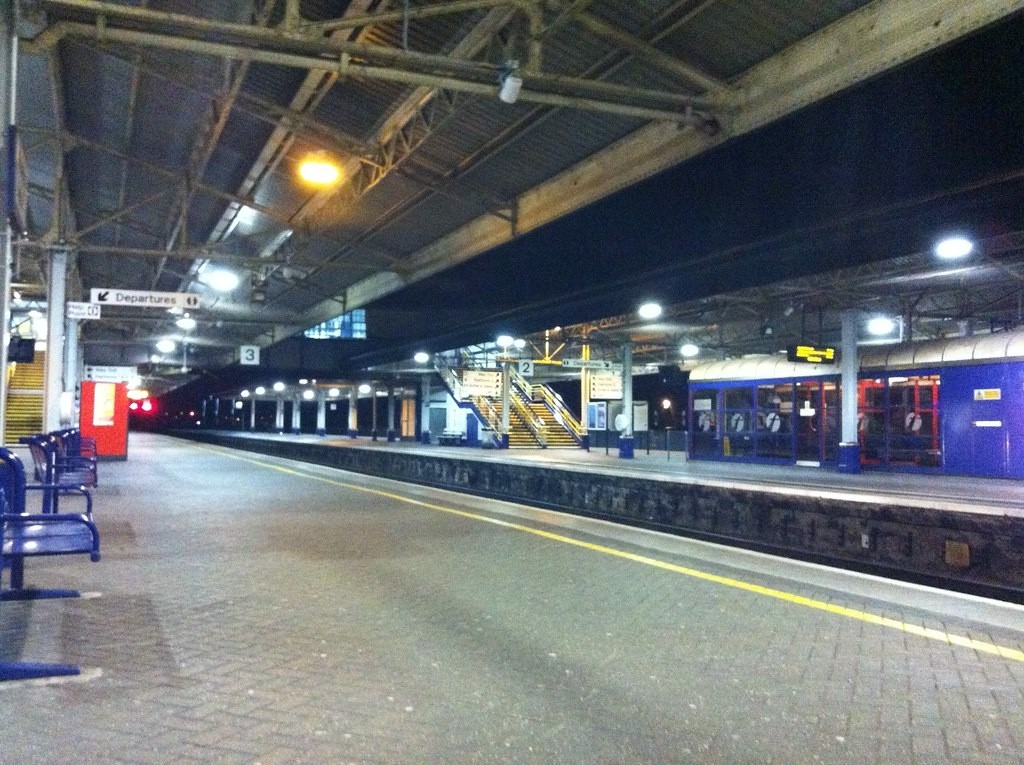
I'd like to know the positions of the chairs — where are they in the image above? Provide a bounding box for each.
[0,427,102,681]
